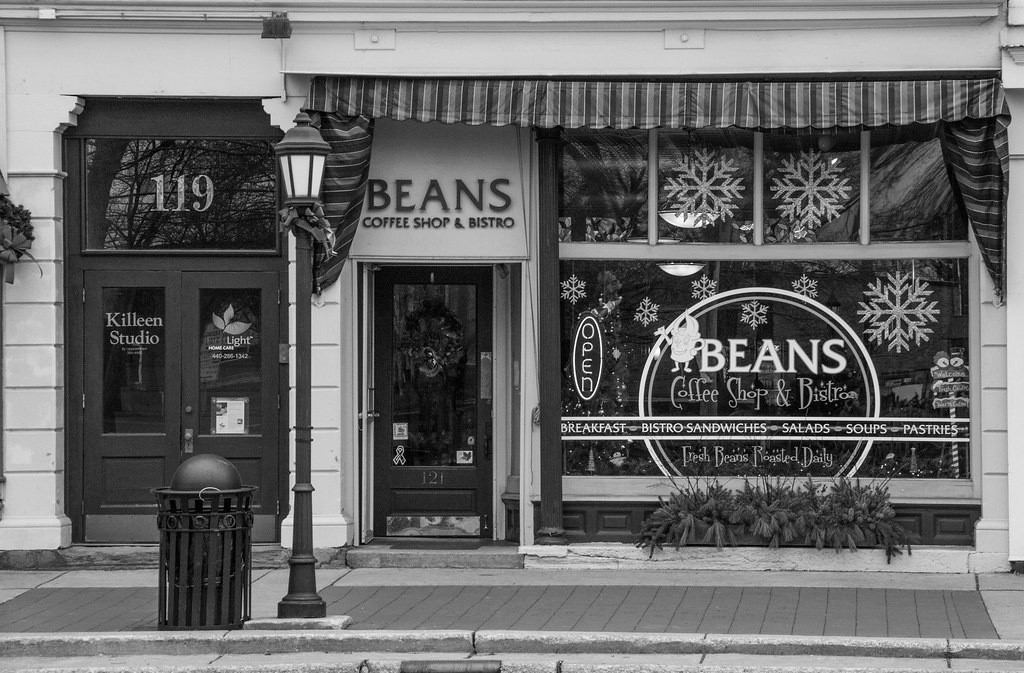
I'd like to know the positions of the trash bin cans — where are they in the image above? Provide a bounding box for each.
[149,453,260,632]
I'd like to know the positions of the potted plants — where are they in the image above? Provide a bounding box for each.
[636,458,922,563]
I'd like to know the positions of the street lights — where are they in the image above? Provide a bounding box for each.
[273,115,331,622]
